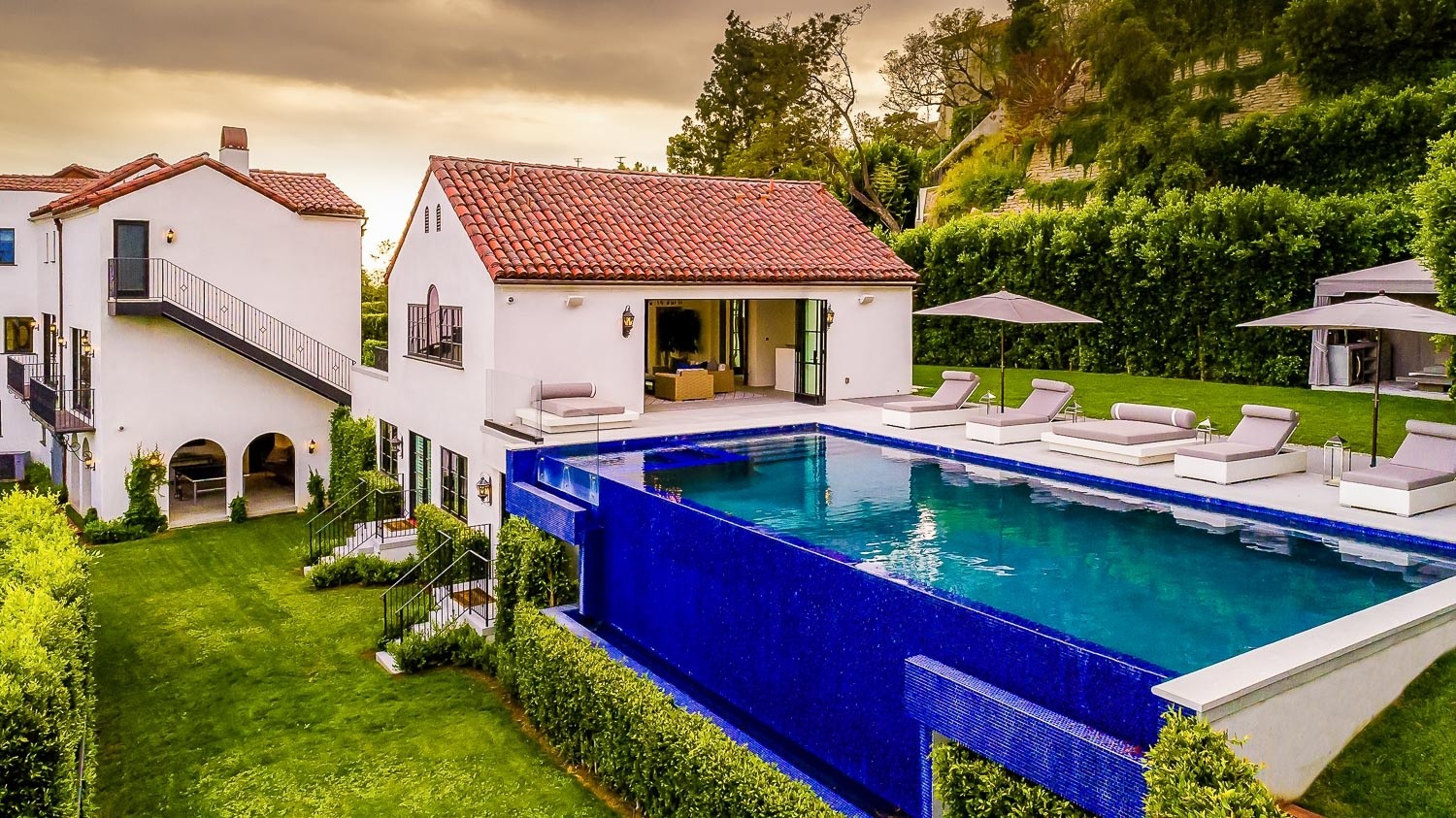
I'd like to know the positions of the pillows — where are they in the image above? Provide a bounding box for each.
[679,361,708,370]
[667,356,689,373]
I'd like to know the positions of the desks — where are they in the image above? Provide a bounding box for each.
[172,462,250,505]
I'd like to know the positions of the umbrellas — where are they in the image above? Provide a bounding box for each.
[1235,292,1456,467]
[912,288,1104,412]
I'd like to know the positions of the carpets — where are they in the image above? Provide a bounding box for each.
[644,391,766,405]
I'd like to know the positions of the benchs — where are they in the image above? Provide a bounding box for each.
[1176,404,1306,485]
[883,371,985,430]
[965,378,1075,445]
[516,382,639,434]
[1339,420,1456,517]
[1039,402,1209,466]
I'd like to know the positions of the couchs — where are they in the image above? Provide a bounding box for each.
[652,359,735,401]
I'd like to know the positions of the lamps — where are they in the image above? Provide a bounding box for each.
[80,334,88,346]
[309,439,316,454]
[83,342,95,358]
[827,304,834,329]
[476,471,492,506]
[30,318,39,331]
[166,228,174,243]
[58,336,67,349]
[389,435,403,459]
[50,324,60,336]
[83,451,95,471]
[622,305,635,338]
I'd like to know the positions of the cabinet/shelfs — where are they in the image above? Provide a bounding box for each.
[1328,342,1377,386]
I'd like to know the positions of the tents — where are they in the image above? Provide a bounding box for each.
[1309,256,1456,387]
